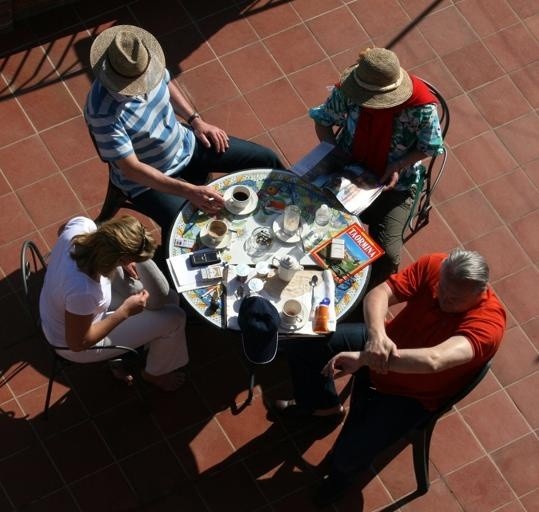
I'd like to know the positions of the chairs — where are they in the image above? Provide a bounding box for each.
[339,357,495,494]
[84,111,209,268]
[20,240,149,421]
[401,76,450,249]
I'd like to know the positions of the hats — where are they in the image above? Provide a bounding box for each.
[237,295,283,365]
[339,46,413,111]
[89,23,167,99]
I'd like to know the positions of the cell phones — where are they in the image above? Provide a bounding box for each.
[190,251,222,267]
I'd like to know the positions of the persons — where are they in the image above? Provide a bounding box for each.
[36,212,190,394]
[83,65,286,238]
[277,249,508,511]
[285,68,445,284]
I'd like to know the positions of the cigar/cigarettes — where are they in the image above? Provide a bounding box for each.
[208,197,215,201]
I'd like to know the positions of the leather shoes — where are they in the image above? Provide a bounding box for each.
[273,397,346,421]
[313,467,355,506]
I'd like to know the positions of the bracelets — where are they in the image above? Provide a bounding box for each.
[186,112,200,124]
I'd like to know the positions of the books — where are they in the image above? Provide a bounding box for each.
[289,138,389,217]
[307,223,387,284]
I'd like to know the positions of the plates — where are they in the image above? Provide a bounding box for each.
[199,185,334,332]
